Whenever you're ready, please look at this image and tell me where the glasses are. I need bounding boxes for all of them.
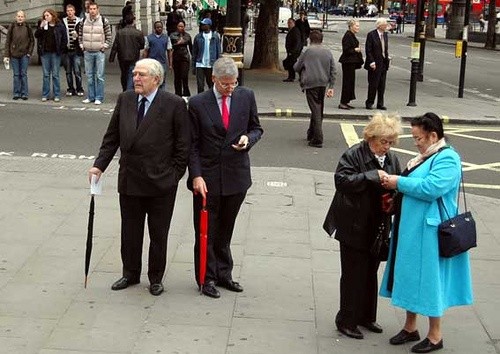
[411,133,431,143]
[217,76,239,89]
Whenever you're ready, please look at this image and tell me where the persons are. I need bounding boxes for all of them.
[79,0,94,21]
[296,12,310,46]
[60,3,85,95]
[108,13,144,91]
[3,9,34,100]
[323,110,402,338]
[190,17,221,93]
[283,17,302,82]
[142,20,173,91]
[338,18,364,108]
[88,58,192,296]
[479,13,484,31]
[167,5,184,35]
[34,8,67,102]
[378,111,474,353]
[116,5,136,28]
[201,4,226,18]
[78,2,113,104]
[169,20,193,103]
[293,29,336,147]
[432,7,450,28]
[166,1,171,10]
[389,11,402,33]
[187,55,264,298]
[122,0,131,14]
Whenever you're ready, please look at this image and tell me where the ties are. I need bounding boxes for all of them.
[380,34,385,58]
[220,94,229,129]
[135,97,146,129]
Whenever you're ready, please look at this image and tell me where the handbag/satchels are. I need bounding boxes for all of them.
[370,214,390,262]
[282,56,297,70]
[437,211,477,258]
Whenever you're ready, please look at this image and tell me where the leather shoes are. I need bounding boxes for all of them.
[197,280,221,299]
[338,103,350,110]
[377,105,387,111]
[346,104,356,109]
[149,279,164,296]
[411,339,444,354]
[360,319,383,335]
[214,277,244,293]
[389,328,421,345]
[110,276,141,290]
[366,104,374,110]
[335,317,364,340]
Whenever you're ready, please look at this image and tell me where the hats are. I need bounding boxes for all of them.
[199,18,212,26]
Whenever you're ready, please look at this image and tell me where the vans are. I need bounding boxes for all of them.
[278,8,292,32]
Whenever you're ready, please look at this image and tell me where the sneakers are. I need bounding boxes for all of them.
[21,97,28,101]
[94,99,102,105]
[308,140,323,148]
[283,77,294,83]
[41,96,49,101]
[77,90,84,97]
[53,96,60,102]
[81,96,94,103]
[13,95,20,100]
[65,90,74,96]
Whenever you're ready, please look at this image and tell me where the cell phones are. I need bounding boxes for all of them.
[236,143,245,147]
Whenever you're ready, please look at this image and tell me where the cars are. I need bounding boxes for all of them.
[307,17,323,32]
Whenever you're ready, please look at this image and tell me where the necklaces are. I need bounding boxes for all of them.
[364,16,389,109]
